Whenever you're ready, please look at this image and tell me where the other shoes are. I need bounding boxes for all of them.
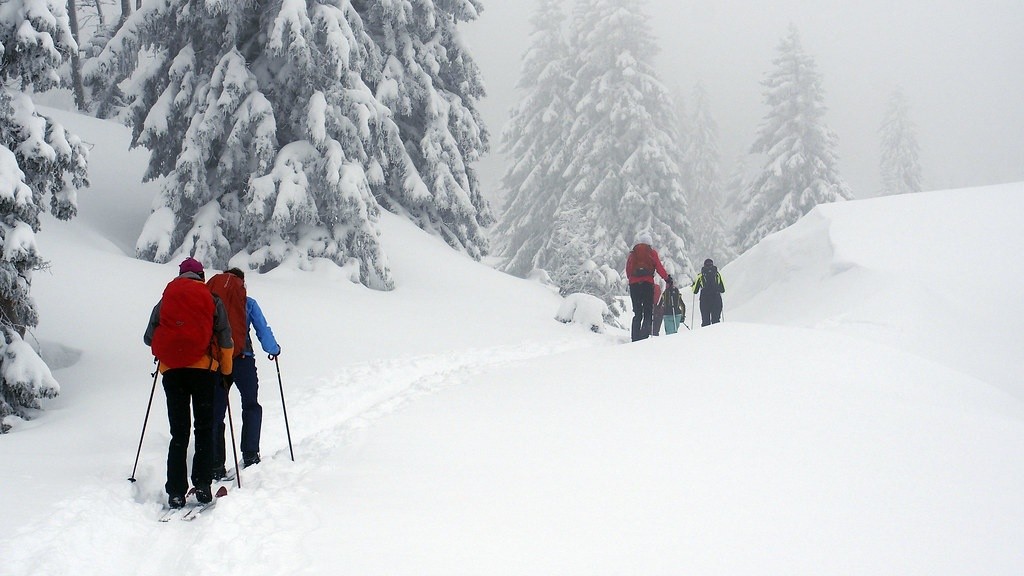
[242,452,259,466]
[213,468,226,480]
[168,493,186,509]
[195,486,212,503]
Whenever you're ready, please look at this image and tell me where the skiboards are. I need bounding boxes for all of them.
[158,485,228,522]
[213,442,261,481]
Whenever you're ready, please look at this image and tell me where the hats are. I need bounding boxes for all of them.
[180,257,204,273]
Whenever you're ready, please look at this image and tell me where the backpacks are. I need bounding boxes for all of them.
[631,242,653,276]
[150,277,215,369]
[701,264,719,294]
[210,273,249,360]
[663,287,680,315]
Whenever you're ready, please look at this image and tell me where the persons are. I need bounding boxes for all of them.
[653,283,683,336]
[144,259,234,508]
[627,236,673,342]
[692,259,725,327]
[212,268,281,480]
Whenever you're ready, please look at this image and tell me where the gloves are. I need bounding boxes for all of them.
[219,342,234,376]
[271,344,281,355]
